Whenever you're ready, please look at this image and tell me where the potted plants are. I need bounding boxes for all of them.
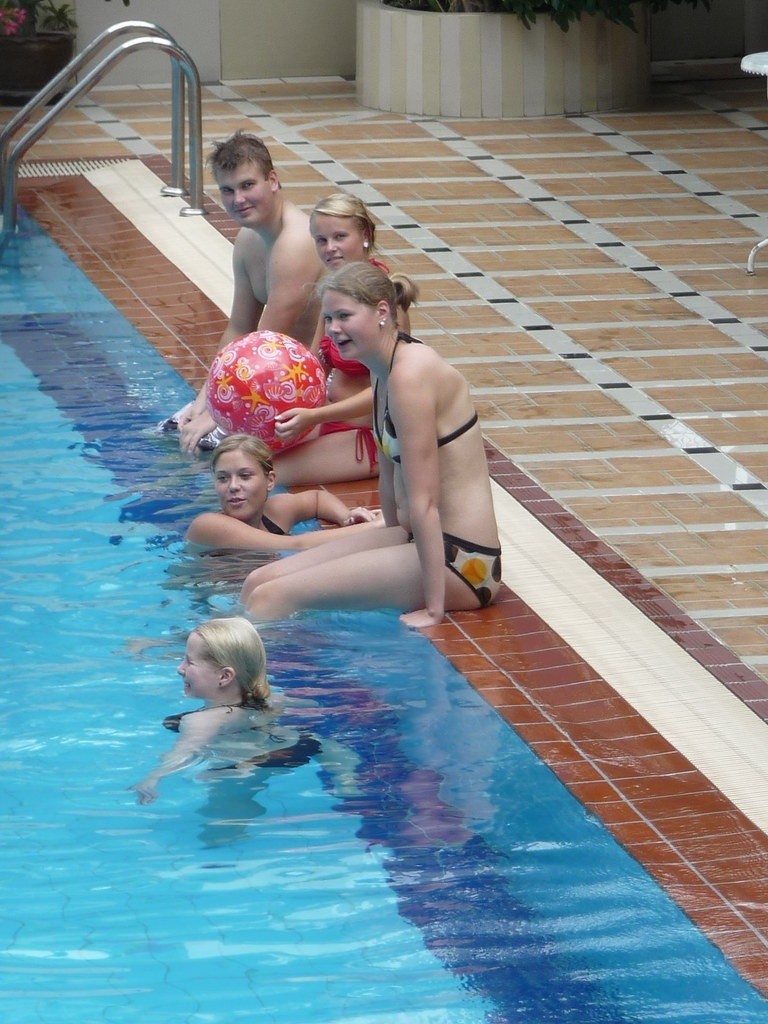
[353,0,669,119]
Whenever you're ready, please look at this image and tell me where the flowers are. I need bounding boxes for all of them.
[0,0,77,37]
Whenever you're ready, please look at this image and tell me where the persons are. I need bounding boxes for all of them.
[184,434,382,554]
[161,129,332,455]
[241,262,503,629]
[206,193,412,486]
[128,617,368,849]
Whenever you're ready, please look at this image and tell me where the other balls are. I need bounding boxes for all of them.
[207,331,331,450]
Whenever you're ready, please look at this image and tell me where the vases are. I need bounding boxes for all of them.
[0,33,75,106]
